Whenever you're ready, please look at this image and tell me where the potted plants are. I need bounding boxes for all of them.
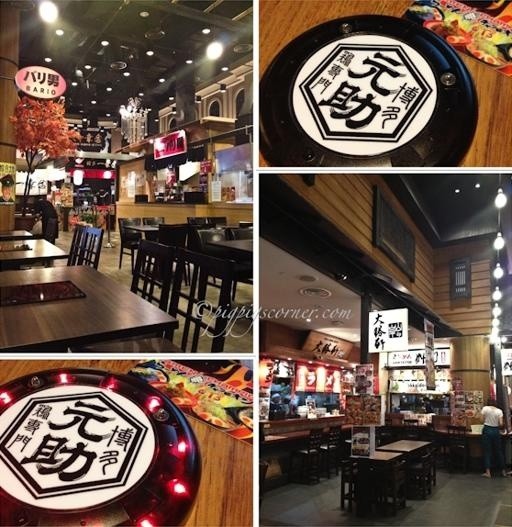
[8,96,83,233]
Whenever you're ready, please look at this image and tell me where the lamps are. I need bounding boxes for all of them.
[206,10,224,60]
[491,260,504,344]
[494,186,508,209]
[119,96,149,147]
[493,230,504,251]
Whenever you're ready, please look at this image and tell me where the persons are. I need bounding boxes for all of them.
[270,393,287,420]
[423,398,434,413]
[0,175,15,203]
[32,196,59,245]
[479,399,507,478]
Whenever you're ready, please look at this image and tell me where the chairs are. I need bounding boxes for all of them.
[0,217,104,272]
[340,420,438,519]
[80,248,235,353]
[288,427,324,485]
[118,217,252,319]
[446,425,469,474]
[309,424,342,480]
[79,237,176,352]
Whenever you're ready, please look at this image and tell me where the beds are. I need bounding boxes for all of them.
[0,266,179,352]
